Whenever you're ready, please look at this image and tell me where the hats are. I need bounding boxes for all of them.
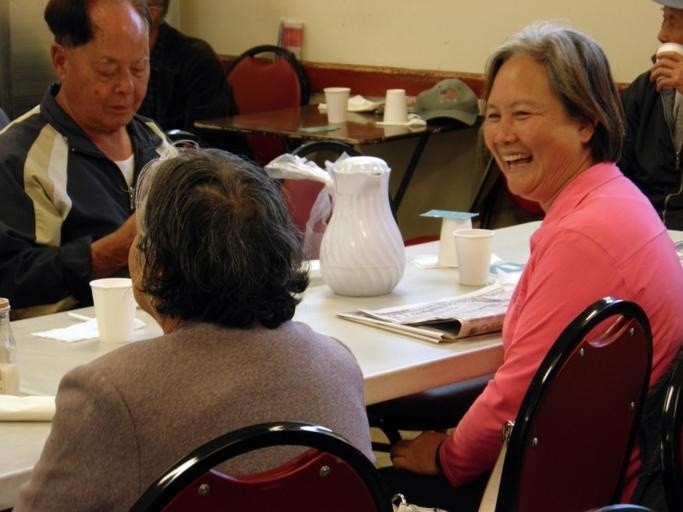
[415,78,480,126]
[652,0,682,10]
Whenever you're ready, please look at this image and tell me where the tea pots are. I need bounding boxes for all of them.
[316,162,404,293]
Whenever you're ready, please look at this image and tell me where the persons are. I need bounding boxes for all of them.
[0,0,181,316]
[377,23,683,512]
[616,0,683,231]
[136,0,260,161]
[11,148,377,512]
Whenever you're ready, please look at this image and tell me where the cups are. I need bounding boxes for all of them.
[325,87,350,125]
[384,89,409,123]
[90,277,136,342]
[438,217,471,269]
[656,42,682,61]
[455,229,496,286]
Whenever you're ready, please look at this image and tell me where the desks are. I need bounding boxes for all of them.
[188,107,483,218]
[1,220,683,512]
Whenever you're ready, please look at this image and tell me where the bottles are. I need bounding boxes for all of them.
[0,297,20,391]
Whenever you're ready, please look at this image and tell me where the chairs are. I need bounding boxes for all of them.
[275,141,360,230]
[631,355,683,512]
[228,46,310,113]
[390,116,504,246]
[393,298,653,512]
[664,181,683,230]
[129,422,394,512]
